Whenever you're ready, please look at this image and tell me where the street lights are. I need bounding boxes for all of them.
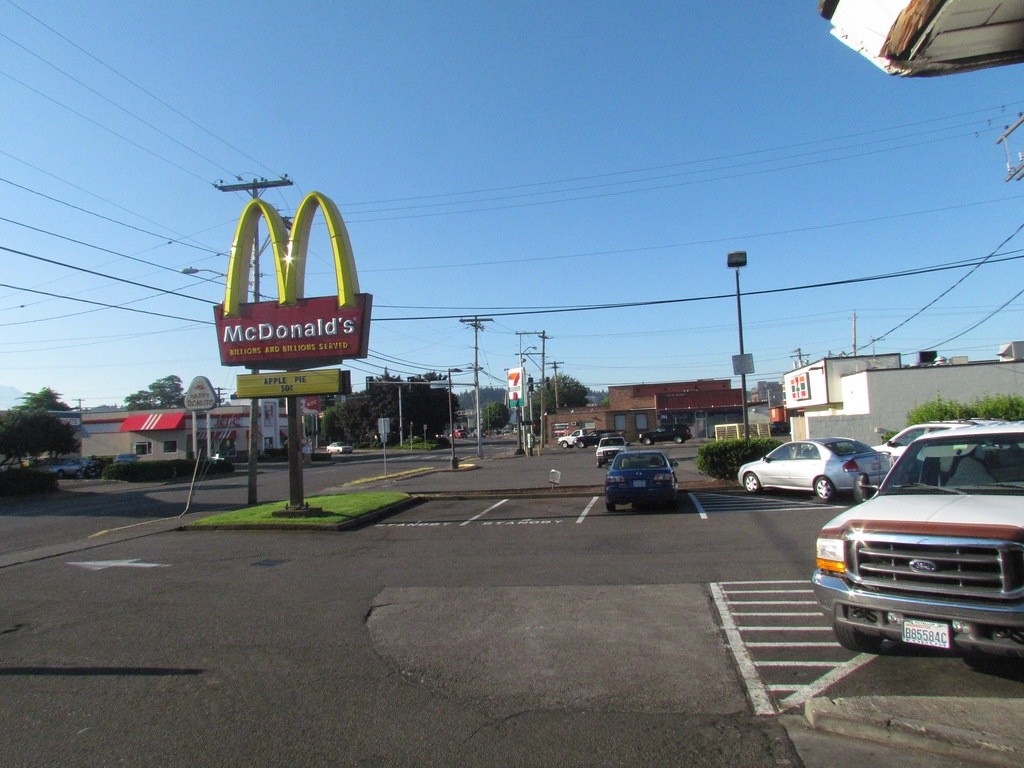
[727,251,749,440]
[448,368,464,469]
[181,266,261,505]
[520,345,538,455]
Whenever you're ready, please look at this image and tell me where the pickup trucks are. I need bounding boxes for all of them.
[557,429,586,449]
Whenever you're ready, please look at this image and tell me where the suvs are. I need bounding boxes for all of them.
[638,423,692,446]
[871,417,1024,464]
[811,425,1024,660]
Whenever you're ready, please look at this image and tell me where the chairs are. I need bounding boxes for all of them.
[945,456,997,486]
[799,448,809,458]
[622,458,629,468]
[650,457,659,468]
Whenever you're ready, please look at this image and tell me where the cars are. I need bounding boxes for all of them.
[593,436,630,469]
[575,429,621,449]
[604,450,679,513]
[24,457,90,480]
[741,437,892,504]
[113,454,141,464]
